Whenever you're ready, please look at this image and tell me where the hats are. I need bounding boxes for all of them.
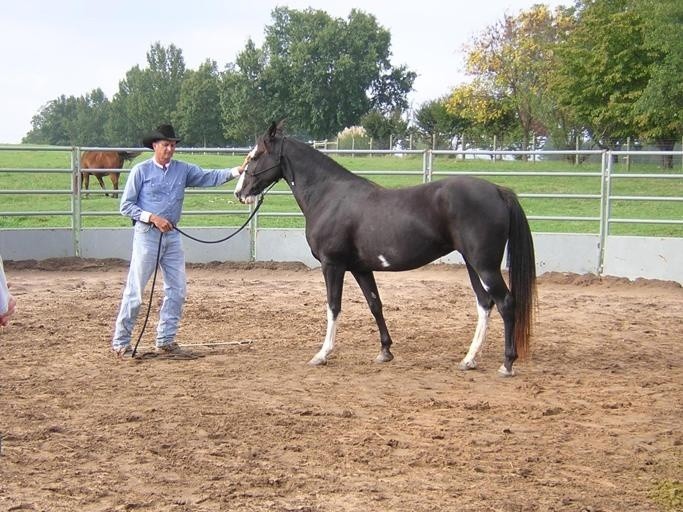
[141,122,182,150]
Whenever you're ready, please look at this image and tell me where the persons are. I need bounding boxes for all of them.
[113,124,251,363]
[0,256,18,328]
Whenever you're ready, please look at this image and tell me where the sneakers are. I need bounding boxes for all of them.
[113,344,142,361]
[153,340,181,354]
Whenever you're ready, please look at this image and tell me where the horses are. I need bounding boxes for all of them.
[233,117,538,378]
[75,150,142,198]
